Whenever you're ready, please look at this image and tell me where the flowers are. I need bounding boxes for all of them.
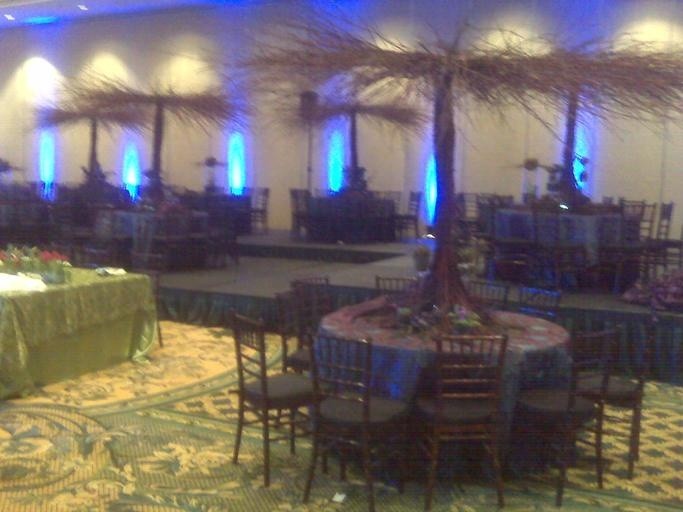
[386,298,481,335]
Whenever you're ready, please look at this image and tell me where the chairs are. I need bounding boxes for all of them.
[515,282,564,323]
[301,330,406,511]
[416,333,510,511]
[0,181,683,306]
[131,265,166,349]
[517,321,624,507]
[271,282,314,428]
[222,306,313,486]
[291,274,332,335]
[574,304,659,482]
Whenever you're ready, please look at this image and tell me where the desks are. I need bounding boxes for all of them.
[0,254,151,399]
